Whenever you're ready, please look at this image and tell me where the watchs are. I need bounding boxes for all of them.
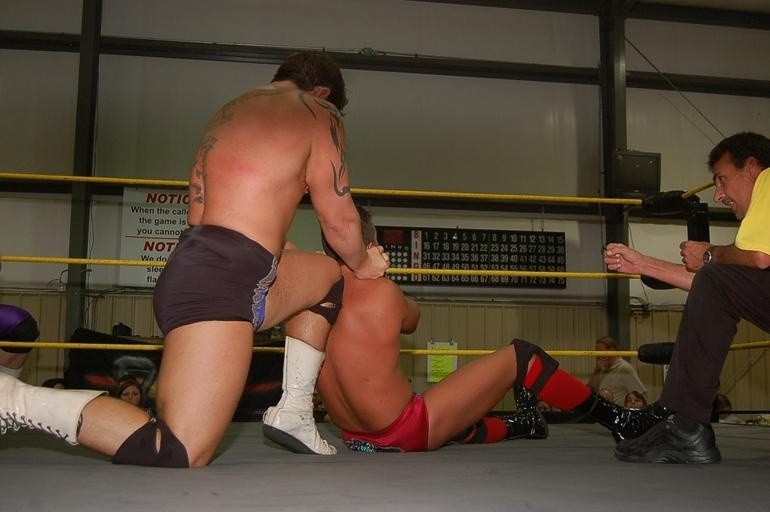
[704,245,718,263]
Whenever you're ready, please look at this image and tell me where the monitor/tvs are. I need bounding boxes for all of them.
[611,149,662,197]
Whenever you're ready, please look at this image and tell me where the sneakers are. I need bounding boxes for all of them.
[612,422,723,466]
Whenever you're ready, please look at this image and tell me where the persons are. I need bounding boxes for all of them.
[590,337,647,409]
[718,394,731,412]
[117,380,145,406]
[604,133,768,464]
[315,206,673,452]
[1,49,390,467]
[43,379,65,389]
[537,400,552,413]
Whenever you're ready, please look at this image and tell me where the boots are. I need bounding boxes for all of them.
[0,372,109,447]
[502,384,550,440]
[576,383,672,445]
[261,336,338,456]
